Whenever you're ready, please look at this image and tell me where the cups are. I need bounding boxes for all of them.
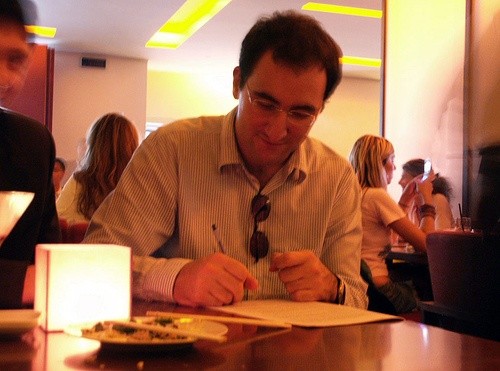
[456,217,472,232]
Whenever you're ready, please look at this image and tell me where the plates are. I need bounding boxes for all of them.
[0,309,40,337]
[82,314,228,348]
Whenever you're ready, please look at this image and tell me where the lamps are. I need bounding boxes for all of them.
[33,244,131,332]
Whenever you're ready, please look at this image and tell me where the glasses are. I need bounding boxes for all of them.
[244,82,322,130]
[250,192,272,263]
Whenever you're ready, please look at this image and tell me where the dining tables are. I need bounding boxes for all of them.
[0,296,500,371]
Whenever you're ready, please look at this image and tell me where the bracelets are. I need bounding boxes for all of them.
[419,204,436,221]
[399,202,410,208]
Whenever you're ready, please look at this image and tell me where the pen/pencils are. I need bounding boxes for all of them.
[211,223,244,304]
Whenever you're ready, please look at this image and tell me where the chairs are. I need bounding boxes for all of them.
[420,233,500,342]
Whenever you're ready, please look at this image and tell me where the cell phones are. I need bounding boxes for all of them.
[421,158,432,182]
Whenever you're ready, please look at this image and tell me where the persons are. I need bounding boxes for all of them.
[56,112,138,225]
[0,0,61,309]
[80,10,369,310]
[349,134,436,314]
[396,159,456,242]
[52,158,65,200]
